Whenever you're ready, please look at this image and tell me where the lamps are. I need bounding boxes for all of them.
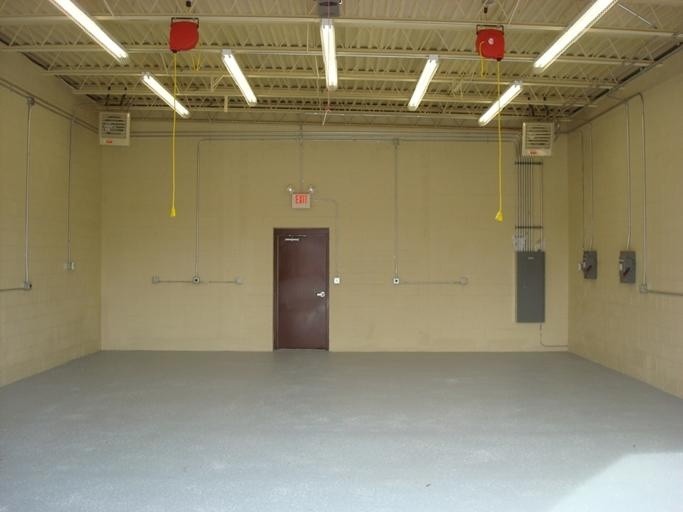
[219,50,257,107]
[51,1,129,67]
[531,0,616,76]
[139,73,191,119]
[319,17,338,93]
[406,56,440,112]
[477,81,522,125]
